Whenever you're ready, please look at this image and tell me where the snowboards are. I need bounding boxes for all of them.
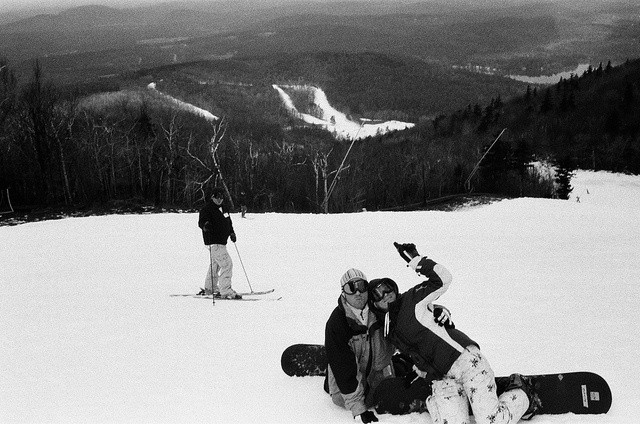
[374,372,612,414]
[281,344,328,377]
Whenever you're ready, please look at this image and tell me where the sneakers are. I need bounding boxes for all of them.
[219,291,237,300]
[505,374,544,421]
[205,289,220,295]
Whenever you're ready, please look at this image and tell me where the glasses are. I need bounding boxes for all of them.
[341,279,372,295]
[369,281,393,303]
[212,193,224,200]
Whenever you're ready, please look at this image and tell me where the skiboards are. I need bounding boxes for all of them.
[169,289,282,301]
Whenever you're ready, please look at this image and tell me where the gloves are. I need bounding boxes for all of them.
[354,409,379,424]
[205,223,212,231]
[428,303,454,327]
[230,233,236,242]
[393,241,421,264]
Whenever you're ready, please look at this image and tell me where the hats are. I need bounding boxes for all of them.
[340,268,369,287]
[370,277,399,303]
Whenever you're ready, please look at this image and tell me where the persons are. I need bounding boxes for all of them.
[575,195,582,203]
[323,268,398,424]
[368,241,543,424]
[240,192,249,220]
[198,189,243,300]
